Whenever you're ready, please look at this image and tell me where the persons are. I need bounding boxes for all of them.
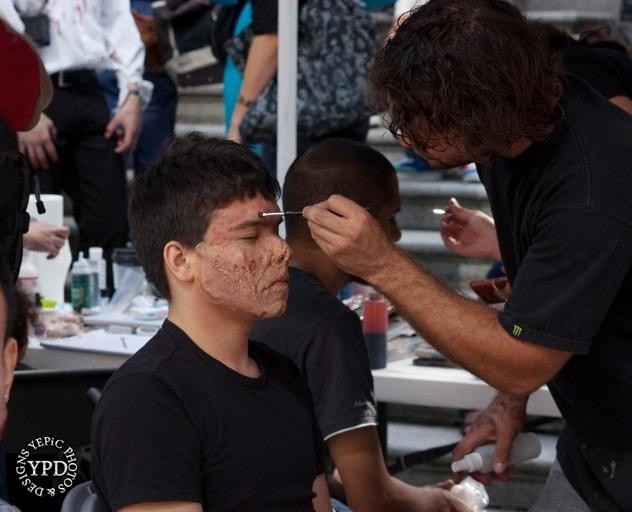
[1,1,632,511]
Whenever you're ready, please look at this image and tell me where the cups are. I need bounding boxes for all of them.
[18,264,42,337]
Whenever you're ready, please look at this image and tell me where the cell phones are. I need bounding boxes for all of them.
[471,277,509,304]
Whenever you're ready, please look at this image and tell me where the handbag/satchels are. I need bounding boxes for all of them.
[225,0,387,144]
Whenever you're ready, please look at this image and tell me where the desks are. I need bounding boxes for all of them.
[371,355,565,478]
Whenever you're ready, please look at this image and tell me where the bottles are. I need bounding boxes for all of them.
[71,246,107,316]
[362,292,388,370]
[451,432,541,475]
